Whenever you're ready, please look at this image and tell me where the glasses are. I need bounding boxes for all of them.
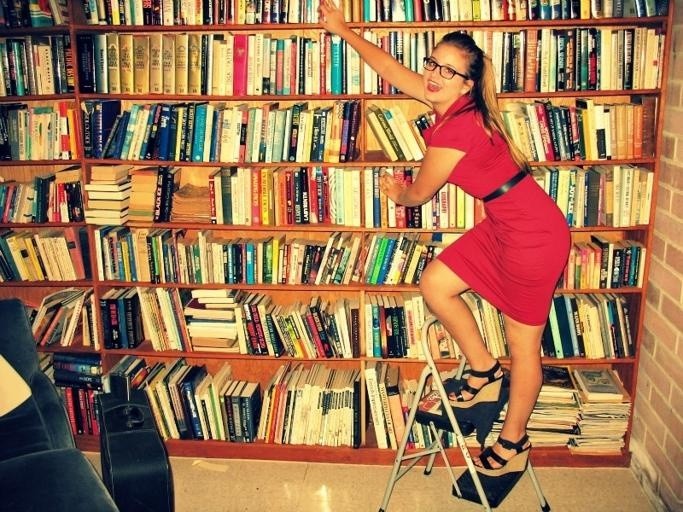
[423,57,470,81]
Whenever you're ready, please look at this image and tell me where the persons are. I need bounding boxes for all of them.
[317,0,571,477]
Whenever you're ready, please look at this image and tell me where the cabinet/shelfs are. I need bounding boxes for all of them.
[0,0,675,469]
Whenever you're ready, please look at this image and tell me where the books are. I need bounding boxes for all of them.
[92,226,467,285]
[365,104,438,162]
[362,26,666,97]
[556,234,646,289]
[23,286,100,351]
[525,163,654,229]
[0,0,70,29]
[0,225,94,281]
[0,103,79,161]
[364,293,510,361]
[0,162,85,225]
[540,293,634,360]
[98,286,359,360]
[364,0,674,22]
[366,361,633,457]
[362,164,486,232]
[37,351,103,435]
[84,164,362,226]
[0,31,76,98]
[76,28,364,97]
[73,0,361,26]
[80,99,362,165]
[501,94,655,162]
[107,355,360,449]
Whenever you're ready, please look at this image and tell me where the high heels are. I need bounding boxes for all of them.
[447,360,503,408]
[472,435,532,476]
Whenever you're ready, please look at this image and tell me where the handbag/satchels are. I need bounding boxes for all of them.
[97,387,176,512]
